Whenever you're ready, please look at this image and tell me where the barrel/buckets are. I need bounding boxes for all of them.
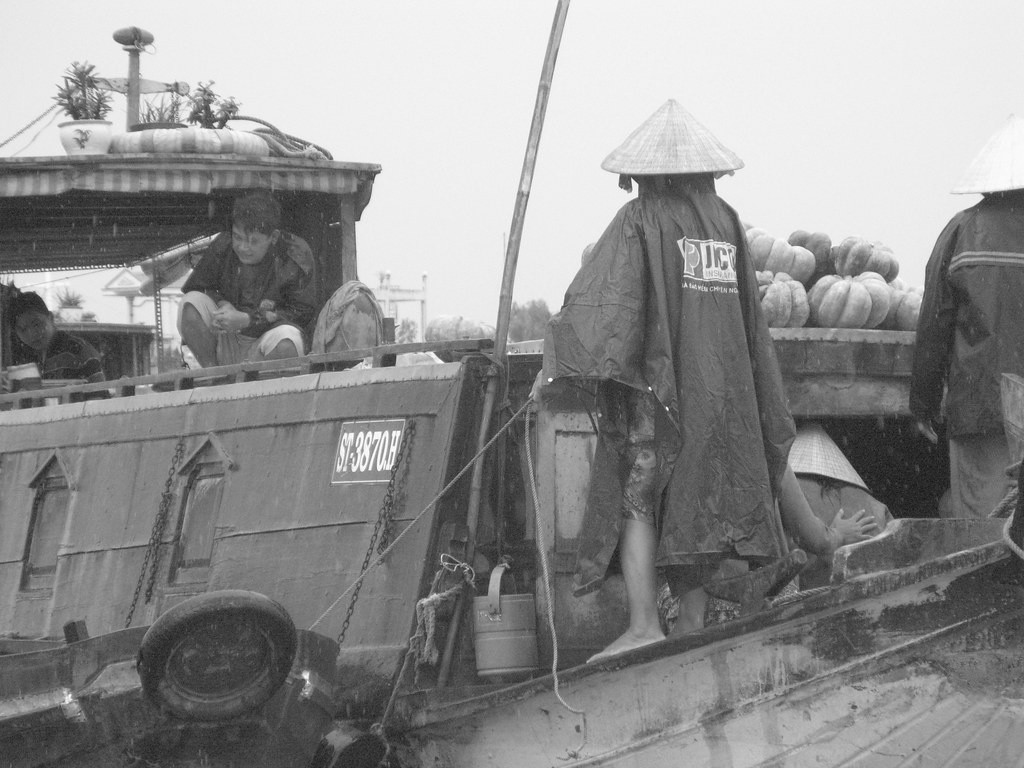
[470,562,540,677]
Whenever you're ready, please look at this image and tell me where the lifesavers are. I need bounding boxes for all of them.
[110,125,271,157]
[136,588,298,722]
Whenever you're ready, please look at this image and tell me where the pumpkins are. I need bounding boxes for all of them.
[506,294,556,343]
[742,226,923,330]
[423,316,497,362]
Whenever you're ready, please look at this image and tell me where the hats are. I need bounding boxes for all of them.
[950,109,1024,195]
[600,99,745,175]
[788,422,874,494]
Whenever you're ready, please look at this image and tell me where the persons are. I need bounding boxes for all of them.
[909,155,1024,519]
[657,421,896,637]
[0,291,111,407]
[176,192,320,388]
[527,99,797,665]
